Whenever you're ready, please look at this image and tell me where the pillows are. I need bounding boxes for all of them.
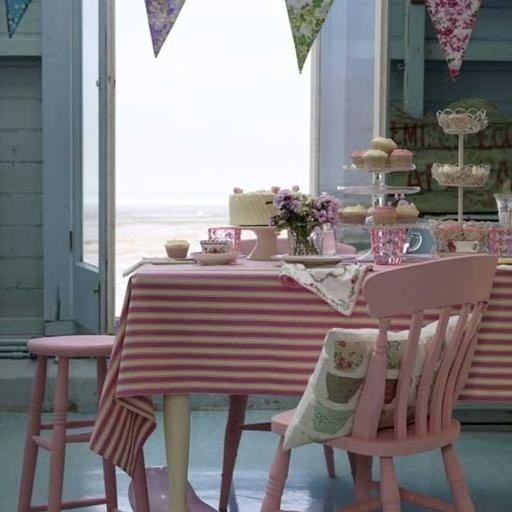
[282,312,477,451]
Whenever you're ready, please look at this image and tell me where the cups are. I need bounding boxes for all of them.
[448,240,479,253]
[201,240,229,253]
[370,227,422,265]
[320,229,336,255]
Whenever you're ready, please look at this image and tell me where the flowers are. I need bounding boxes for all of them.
[266,186,341,255]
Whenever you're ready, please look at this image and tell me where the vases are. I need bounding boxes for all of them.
[494,190,512,236]
[286,222,326,257]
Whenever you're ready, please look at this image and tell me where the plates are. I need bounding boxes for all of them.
[437,108,486,135]
[232,225,287,261]
[433,162,490,187]
[190,251,238,265]
[429,219,493,252]
[343,164,416,172]
[272,253,355,267]
[337,220,432,229]
[337,186,420,195]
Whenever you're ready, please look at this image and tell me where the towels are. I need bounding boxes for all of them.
[278,263,373,316]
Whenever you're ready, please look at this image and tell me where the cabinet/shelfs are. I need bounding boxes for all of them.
[374,3,512,219]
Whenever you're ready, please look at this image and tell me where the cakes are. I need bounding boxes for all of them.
[371,137,399,159]
[229,186,299,225]
[389,149,413,169]
[344,204,367,224]
[362,149,387,169]
[366,206,373,216]
[350,149,365,167]
[337,208,349,224]
[396,199,420,224]
[374,207,397,227]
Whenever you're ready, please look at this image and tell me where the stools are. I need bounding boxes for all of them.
[17,334,122,508]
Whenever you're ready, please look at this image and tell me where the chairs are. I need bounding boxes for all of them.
[256,253,500,507]
